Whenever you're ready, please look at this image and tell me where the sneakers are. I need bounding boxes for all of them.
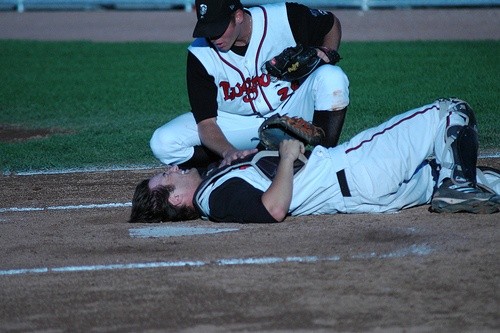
[431,176,500,214]
[476,166,500,196]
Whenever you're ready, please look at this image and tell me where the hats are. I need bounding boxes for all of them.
[193,0,240,39]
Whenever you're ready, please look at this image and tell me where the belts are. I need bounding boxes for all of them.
[336,169,352,197]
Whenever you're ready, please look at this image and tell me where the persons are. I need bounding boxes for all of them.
[150,0,350,178]
[128,95,500,223]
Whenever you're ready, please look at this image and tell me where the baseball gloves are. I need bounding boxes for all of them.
[258,113,325,150]
[265,44,344,82]
